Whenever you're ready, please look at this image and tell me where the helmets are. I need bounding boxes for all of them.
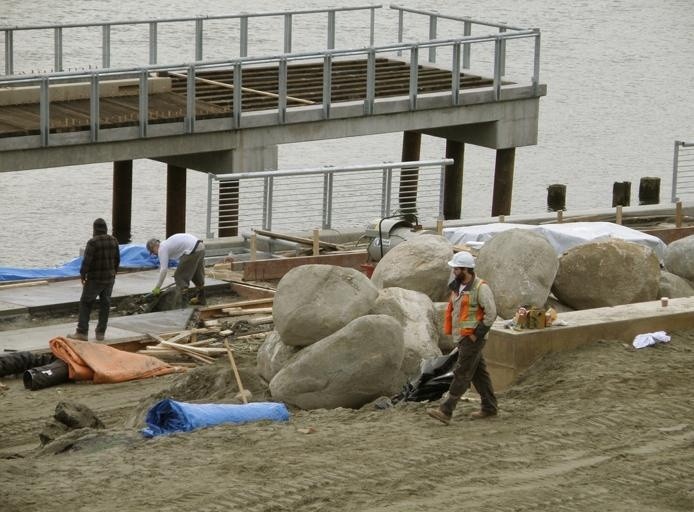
[448,251,475,268]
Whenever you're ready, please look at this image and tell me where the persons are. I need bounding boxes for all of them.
[66,217,120,342]
[146,233,207,306]
[424,251,499,426]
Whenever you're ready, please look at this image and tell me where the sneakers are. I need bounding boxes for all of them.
[428,409,450,425]
[66,333,88,341]
[471,411,497,419]
[96,334,104,340]
[189,298,206,305]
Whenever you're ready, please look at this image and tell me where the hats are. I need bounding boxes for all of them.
[147,239,158,255]
